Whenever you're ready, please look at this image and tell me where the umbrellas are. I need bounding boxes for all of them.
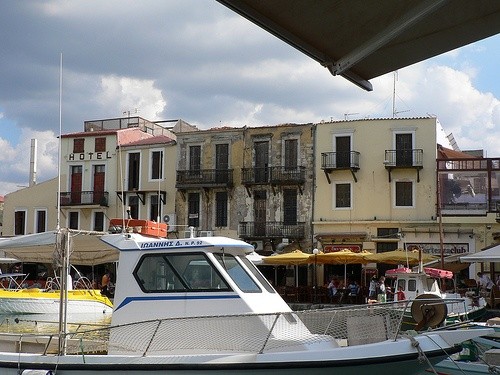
[308,248,373,306]
[188,251,270,290]
[364,247,440,272]
[261,250,315,302]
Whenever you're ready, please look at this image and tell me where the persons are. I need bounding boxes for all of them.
[477,272,497,292]
[101,270,114,294]
[378,274,393,303]
[327,279,362,304]
[369,272,378,302]
[391,285,406,300]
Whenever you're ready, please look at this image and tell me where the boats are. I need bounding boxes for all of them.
[0,53,499,375]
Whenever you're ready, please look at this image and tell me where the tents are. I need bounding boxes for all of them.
[460,244,499,263]
